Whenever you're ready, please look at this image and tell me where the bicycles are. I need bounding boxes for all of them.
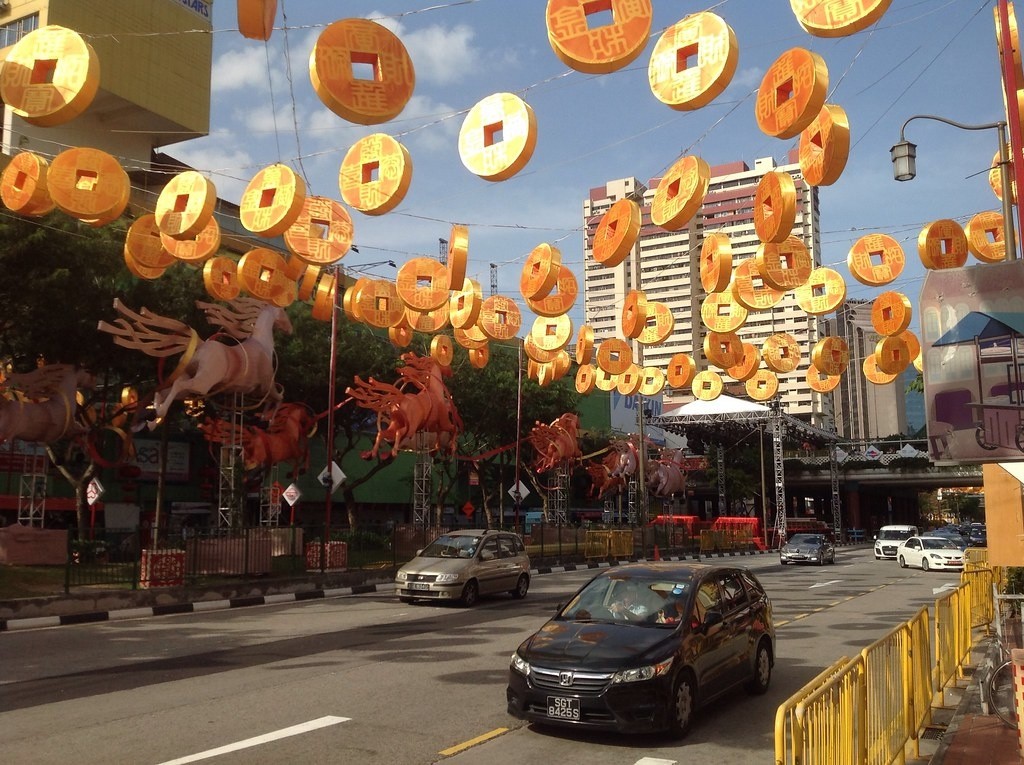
[986,657,1019,731]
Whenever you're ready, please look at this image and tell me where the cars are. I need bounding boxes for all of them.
[926,522,987,547]
[778,533,835,566]
[896,535,964,572]
[505,560,777,741]
[393,527,533,603]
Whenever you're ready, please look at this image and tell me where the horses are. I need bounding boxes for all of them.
[1,296,687,503]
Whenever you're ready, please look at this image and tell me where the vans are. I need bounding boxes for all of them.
[873,524,919,562]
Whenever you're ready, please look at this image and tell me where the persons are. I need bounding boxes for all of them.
[656,593,700,628]
[607,583,648,622]
